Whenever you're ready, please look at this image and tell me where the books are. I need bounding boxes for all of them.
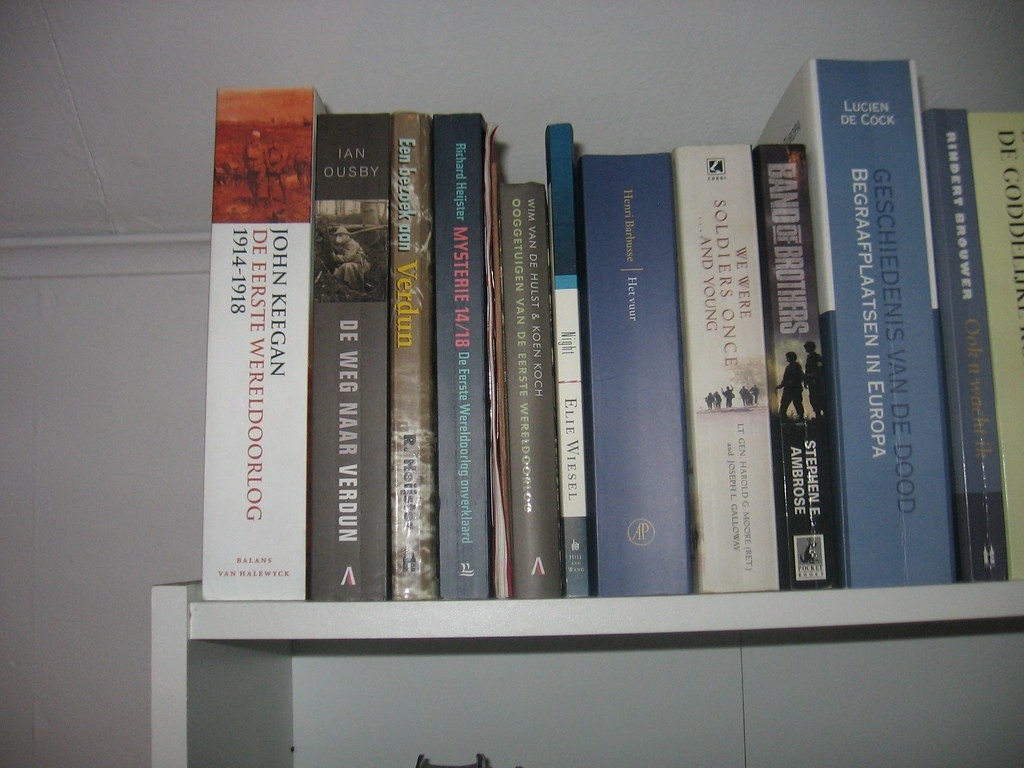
[198,53,1024,599]
